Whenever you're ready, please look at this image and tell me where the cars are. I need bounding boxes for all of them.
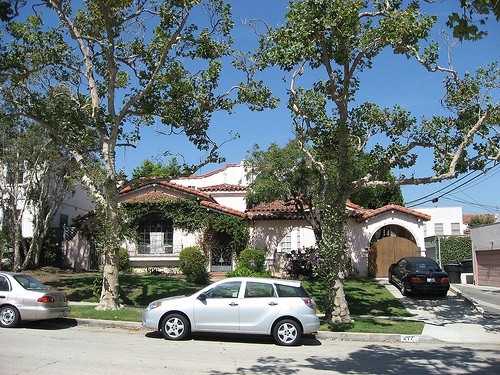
[388,256,450,296]
[142,276,321,345]
[0,272,71,328]
